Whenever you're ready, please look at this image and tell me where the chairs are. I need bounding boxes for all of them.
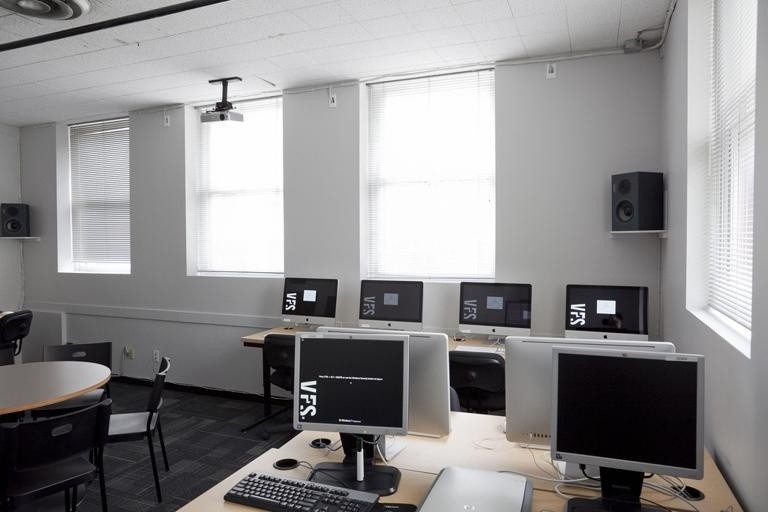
[0,306,178,511]
[254,332,304,443]
[443,346,509,418]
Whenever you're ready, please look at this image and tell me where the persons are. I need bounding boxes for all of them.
[602,313,625,330]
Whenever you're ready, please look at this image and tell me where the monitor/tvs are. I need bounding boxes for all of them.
[293,332,410,494]
[550,343,705,512]
[318,327,450,463]
[565,283,649,341]
[505,336,676,483]
[458,281,532,339]
[281,276,340,328]
[358,279,424,332]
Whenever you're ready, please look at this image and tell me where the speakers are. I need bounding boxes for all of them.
[0,203,29,237]
[611,171,663,231]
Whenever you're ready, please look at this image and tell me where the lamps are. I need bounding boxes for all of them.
[0,1,93,21]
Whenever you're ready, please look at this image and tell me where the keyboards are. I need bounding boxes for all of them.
[222,472,379,512]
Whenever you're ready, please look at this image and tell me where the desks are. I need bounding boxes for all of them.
[273,407,747,512]
[234,323,507,435]
[173,446,751,511]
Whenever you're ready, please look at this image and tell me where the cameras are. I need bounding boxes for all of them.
[601,317,615,326]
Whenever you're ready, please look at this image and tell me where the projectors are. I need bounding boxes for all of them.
[200,110,244,123]
